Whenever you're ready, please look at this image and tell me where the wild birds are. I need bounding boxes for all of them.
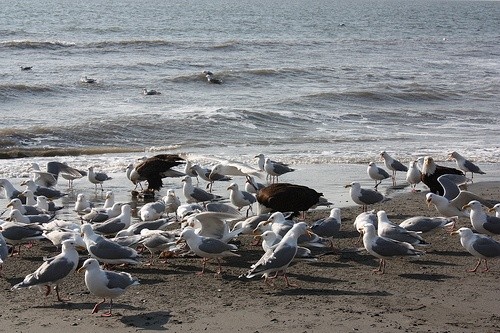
[0,66,500,317]
